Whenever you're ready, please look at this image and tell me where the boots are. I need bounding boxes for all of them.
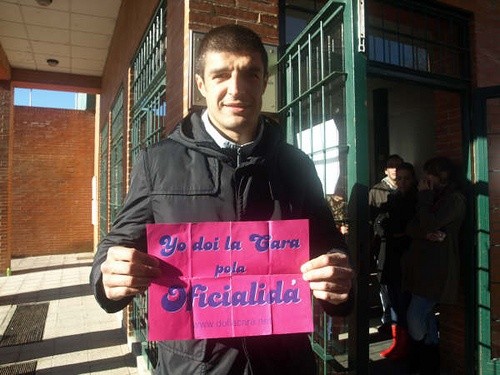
[379,324,440,375]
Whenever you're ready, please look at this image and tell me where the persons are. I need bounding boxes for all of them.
[368,155,474,362]
[90,24,356,375]
